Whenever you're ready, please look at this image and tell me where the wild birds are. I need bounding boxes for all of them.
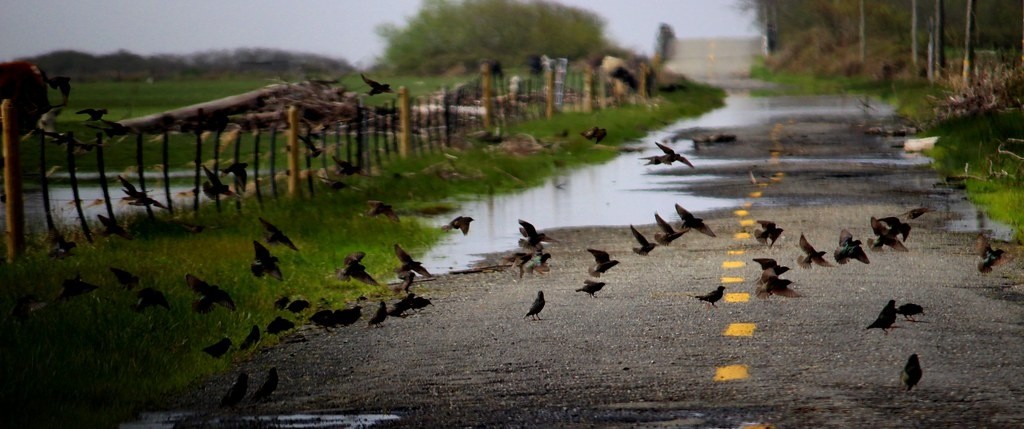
[5,52,1009,414]
